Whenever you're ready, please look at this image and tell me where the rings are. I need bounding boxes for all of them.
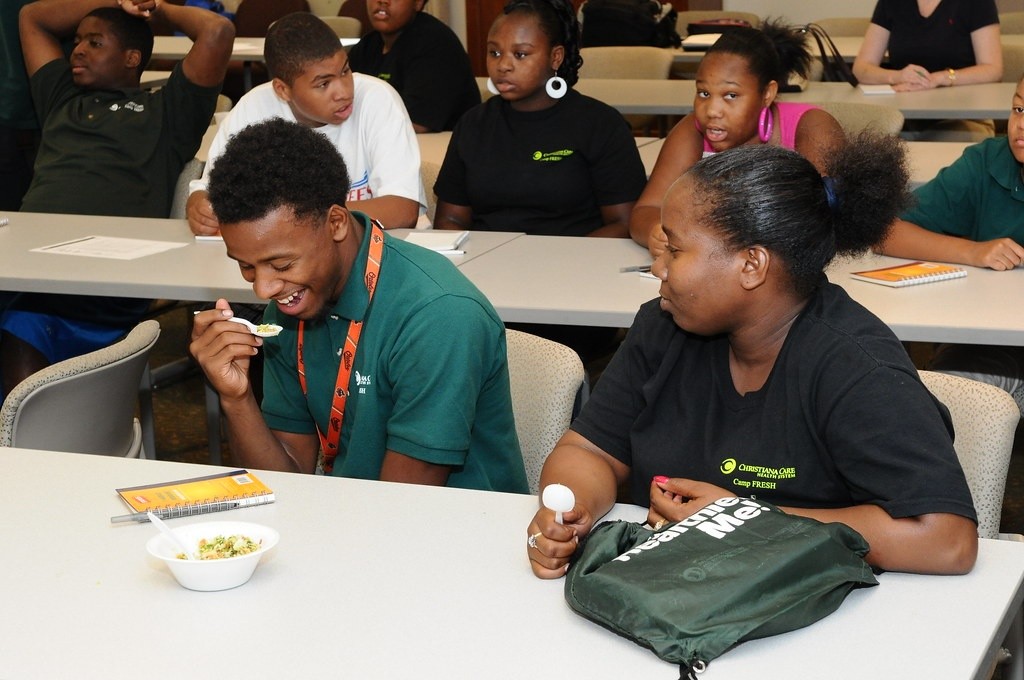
[528,533,542,550]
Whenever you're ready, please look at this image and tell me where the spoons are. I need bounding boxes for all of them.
[194,310,283,336]
[543,483,574,524]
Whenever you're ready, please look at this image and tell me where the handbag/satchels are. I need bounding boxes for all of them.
[806,19,856,87]
[565,495,880,673]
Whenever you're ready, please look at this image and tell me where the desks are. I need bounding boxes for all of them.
[0,35,1024,680]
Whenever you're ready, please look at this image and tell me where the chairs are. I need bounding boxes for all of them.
[169,93,233,221]
[503,326,589,495]
[676,9,761,40]
[804,17,870,83]
[805,96,909,144]
[916,369,1020,539]
[0,320,161,458]
[575,46,674,137]
[321,18,362,53]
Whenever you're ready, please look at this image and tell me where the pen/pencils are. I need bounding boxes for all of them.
[437,250,466,254]
[110,502,241,522]
[621,264,652,273]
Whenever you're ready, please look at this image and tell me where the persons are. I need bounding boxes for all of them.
[872,73,1024,272]
[851,0,1004,145]
[627,17,850,260]
[432,1,647,239]
[344,0,484,136]
[0,1,236,407]
[526,121,977,580]
[188,117,531,495]
[185,12,429,235]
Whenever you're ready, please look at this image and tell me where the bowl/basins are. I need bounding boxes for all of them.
[146,522,280,591]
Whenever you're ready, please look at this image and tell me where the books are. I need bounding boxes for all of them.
[117,468,276,525]
[849,262,968,288]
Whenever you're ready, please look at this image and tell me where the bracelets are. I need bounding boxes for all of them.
[945,68,955,87]
